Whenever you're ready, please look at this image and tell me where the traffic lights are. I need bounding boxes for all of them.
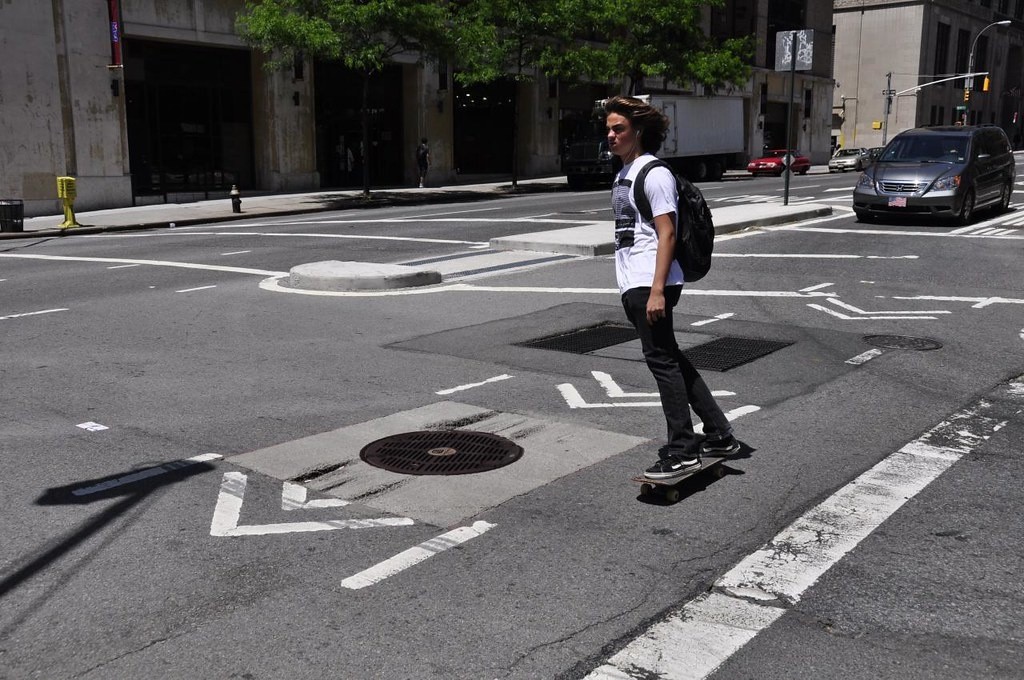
[964,89,969,104]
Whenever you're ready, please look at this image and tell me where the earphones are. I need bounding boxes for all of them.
[636,130,640,135]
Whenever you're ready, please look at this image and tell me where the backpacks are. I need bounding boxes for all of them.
[633,158,715,281]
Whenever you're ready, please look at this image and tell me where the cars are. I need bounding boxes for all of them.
[746,150,811,177]
[828,146,892,172]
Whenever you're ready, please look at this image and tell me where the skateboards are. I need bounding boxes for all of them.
[630,455,728,502]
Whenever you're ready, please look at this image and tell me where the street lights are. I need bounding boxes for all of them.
[964,21,1011,127]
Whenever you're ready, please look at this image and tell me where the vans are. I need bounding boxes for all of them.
[853,126,1016,226]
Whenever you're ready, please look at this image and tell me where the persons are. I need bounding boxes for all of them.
[415,138,432,188]
[604,96,740,481]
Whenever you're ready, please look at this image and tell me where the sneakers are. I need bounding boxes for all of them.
[644,454,702,481]
[697,436,743,457]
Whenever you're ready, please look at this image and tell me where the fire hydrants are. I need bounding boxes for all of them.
[229,185,242,213]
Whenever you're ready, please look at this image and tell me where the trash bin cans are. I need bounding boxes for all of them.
[0,199,24,233]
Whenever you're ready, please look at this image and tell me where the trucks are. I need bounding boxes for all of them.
[548,94,743,191]
